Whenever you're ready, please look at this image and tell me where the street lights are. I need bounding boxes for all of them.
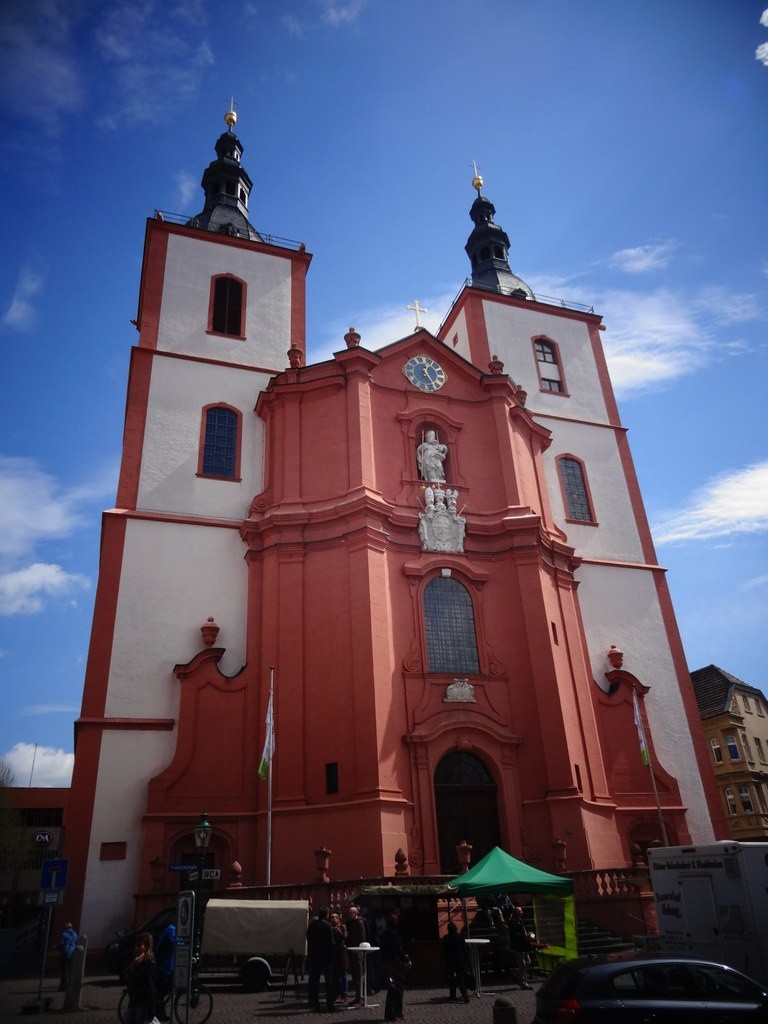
[194,811,214,922]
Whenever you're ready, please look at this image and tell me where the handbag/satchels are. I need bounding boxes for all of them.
[62,955,70,964]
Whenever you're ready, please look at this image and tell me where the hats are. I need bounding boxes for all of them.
[66,923,72,927]
[514,907,523,914]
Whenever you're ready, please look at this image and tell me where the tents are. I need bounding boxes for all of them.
[435,847,581,998]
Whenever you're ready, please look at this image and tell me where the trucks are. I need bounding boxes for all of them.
[106,899,310,984]
[647,839,768,988]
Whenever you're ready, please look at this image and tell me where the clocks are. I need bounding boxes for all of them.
[402,353,449,393]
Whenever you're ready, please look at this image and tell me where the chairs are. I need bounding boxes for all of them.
[641,968,703,998]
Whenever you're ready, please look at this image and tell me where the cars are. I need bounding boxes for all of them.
[530,951,768,1024]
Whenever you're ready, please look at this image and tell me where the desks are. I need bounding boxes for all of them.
[463,939,497,999]
[347,947,379,1010]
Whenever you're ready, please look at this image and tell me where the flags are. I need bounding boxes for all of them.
[257,691,276,783]
[633,691,649,768]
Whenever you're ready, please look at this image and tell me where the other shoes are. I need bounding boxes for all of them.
[326,1006,339,1013]
[515,977,522,986]
[385,1017,406,1024]
[396,1015,406,1019]
[521,983,534,990]
[310,1005,322,1011]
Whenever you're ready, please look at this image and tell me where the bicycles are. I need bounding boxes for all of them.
[118,945,214,1024]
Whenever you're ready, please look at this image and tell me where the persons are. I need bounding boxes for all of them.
[306,906,344,1011]
[442,921,472,1003]
[56,920,78,990]
[509,906,535,991]
[373,904,412,1022]
[346,906,366,1007]
[125,931,163,1024]
[325,909,343,1007]
[689,971,712,1000]
[352,904,381,995]
[417,428,448,482]
[327,913,349,1003]
